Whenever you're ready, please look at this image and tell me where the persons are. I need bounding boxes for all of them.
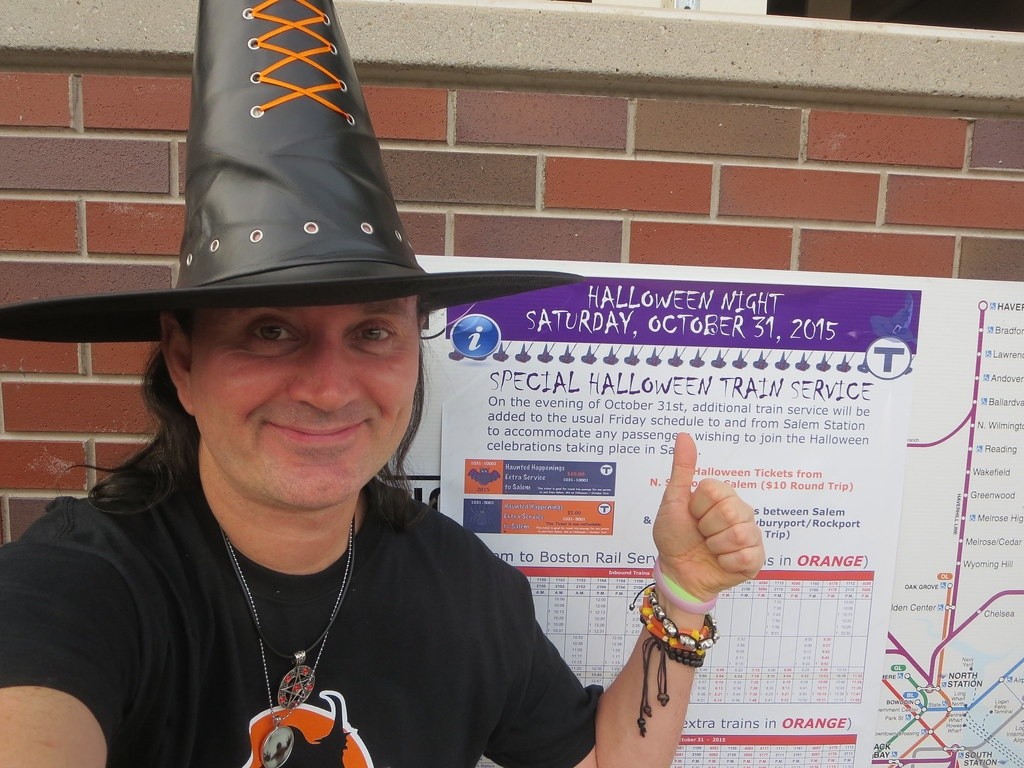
[0,193,764,768]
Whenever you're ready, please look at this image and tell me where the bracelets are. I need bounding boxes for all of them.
[630,556,720,736]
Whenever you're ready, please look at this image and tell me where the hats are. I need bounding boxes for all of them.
[0,0,586,343]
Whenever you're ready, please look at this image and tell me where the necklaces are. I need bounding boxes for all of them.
[225,523,353,768]
[219,516,354,709]
